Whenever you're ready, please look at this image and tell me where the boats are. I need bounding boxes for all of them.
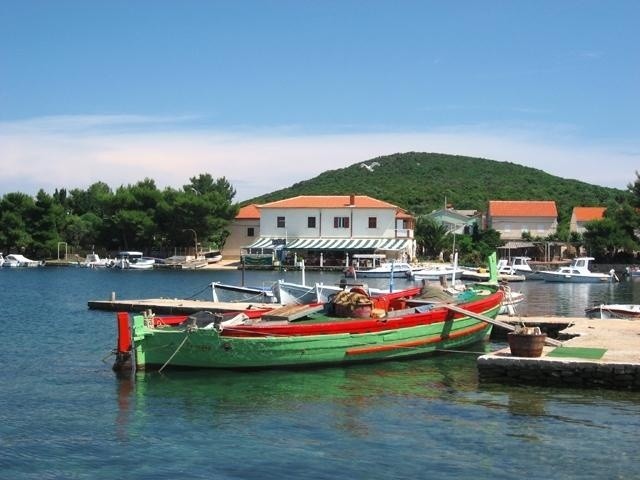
[111,252,155,268]
[540,256,609,284]
[585,304,640,319]
[0,253,42,267]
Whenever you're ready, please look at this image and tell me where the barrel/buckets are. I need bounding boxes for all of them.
[507,327,547,357]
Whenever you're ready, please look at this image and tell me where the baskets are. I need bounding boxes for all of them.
[508,333,547,357]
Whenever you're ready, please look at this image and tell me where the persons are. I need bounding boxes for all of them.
[609,263,640,282]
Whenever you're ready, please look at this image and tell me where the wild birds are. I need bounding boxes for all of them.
[359,161,380,173]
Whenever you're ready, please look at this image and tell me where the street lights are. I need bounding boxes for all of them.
[182,229,197,259]
